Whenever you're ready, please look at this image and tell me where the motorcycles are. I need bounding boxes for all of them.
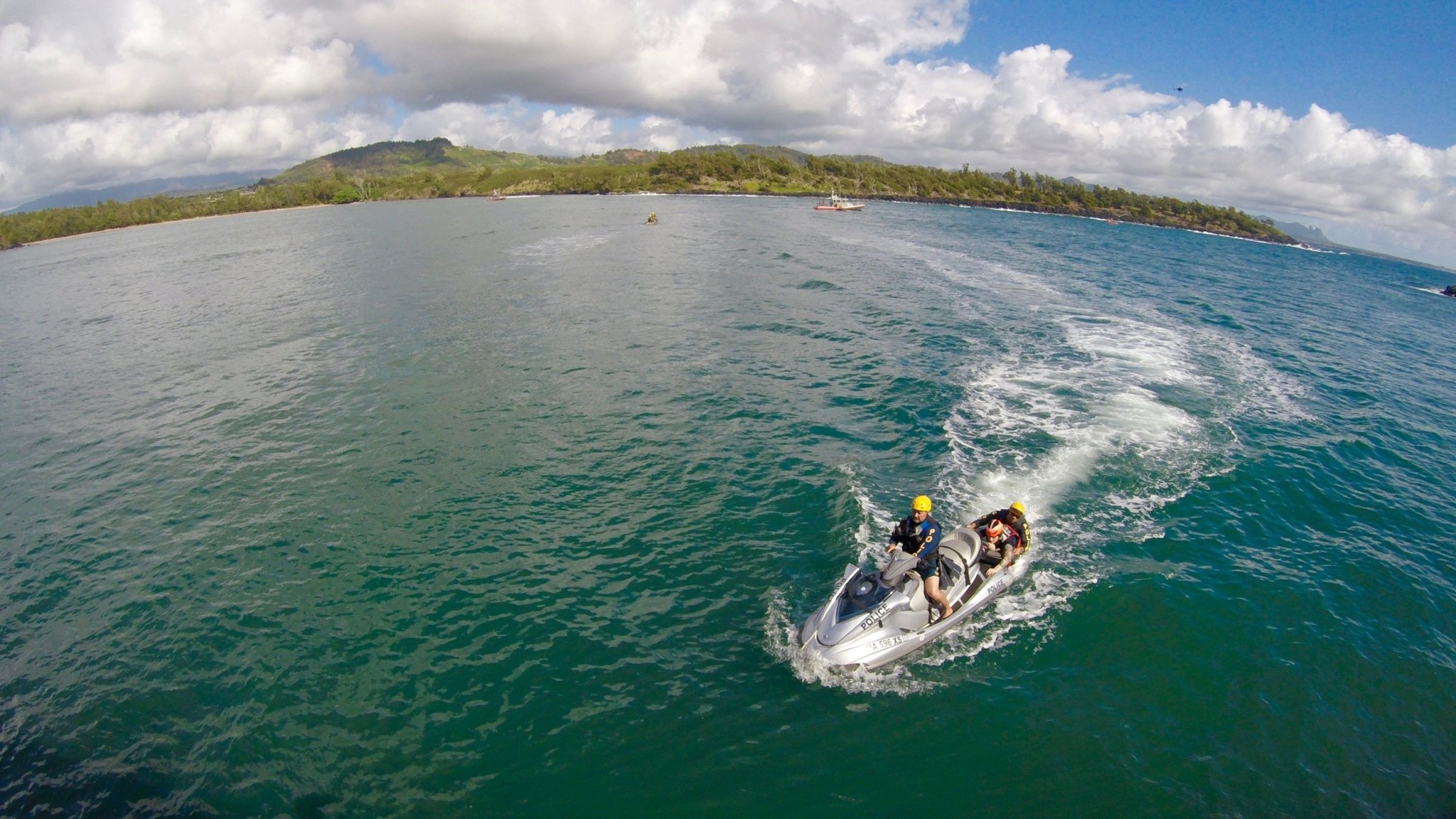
[793,503,1036,676]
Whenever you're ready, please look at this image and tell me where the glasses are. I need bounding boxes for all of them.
[1011,512,1020,519]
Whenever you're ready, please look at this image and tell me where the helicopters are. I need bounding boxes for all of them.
[1173,87,1186,92]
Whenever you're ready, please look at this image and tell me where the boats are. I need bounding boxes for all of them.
[812,190,870,214]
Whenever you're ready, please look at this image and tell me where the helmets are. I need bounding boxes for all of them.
[911,495,932,512]
[1010,502,1024,517]
[986,519,1002,536]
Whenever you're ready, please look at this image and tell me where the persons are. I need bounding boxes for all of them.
[887,495,953,619]
[966,502,1031,576]
